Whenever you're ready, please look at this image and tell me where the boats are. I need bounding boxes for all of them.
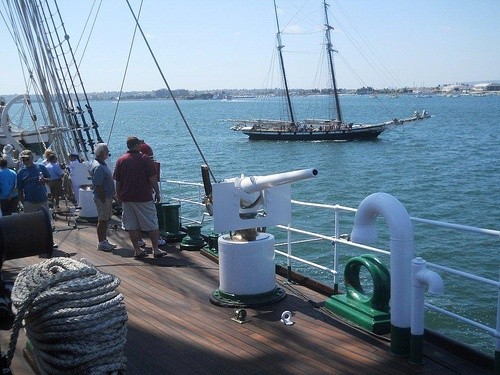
[0,95,57,154]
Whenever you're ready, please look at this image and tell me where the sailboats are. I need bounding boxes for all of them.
[228,0,434,141]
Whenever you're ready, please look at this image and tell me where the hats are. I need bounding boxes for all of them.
[3,144,13,153]
[20,150,32,157]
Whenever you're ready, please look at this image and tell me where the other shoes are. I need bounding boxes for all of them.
[153,249,168,257]
[53,243,58,248]
[134,250,145,260]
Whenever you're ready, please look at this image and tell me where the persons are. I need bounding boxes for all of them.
[90,143,117,251]
[138,143,166,247]
[113,136,168,259]
[0,144,88,247]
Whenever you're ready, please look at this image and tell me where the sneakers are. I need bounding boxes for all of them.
[97,241,117,250]
[138,240,146,247]
[157,240,165,246]
